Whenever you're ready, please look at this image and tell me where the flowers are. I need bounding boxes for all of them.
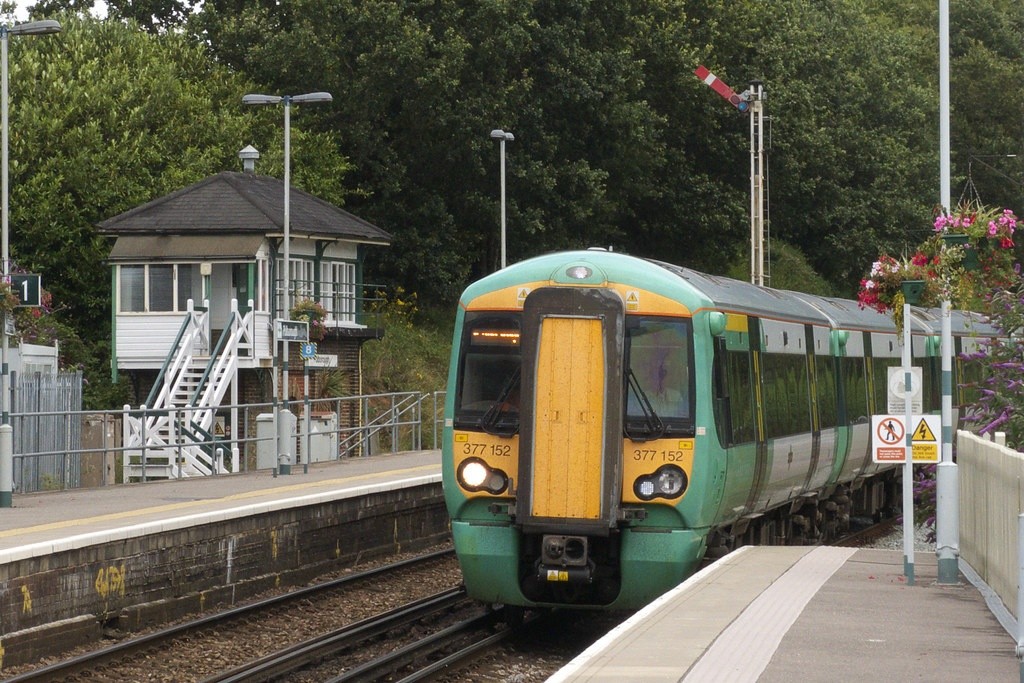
[0,257,52,319]
[857,200,1024,544]
[287,286,419,341]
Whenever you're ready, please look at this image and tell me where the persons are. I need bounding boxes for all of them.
[622,355,687,419]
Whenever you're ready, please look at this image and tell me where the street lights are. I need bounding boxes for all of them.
[240,91,334,475]
[0,20,62,509]
[489,128,515,270]
[898,278,928,588]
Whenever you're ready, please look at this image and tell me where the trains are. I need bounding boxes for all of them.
[442,245,1022,612]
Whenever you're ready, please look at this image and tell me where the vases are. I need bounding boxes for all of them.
[976,236,989,251]
[900,280,926,304]
[940,233,969,249]
[991,238,1001,249]
